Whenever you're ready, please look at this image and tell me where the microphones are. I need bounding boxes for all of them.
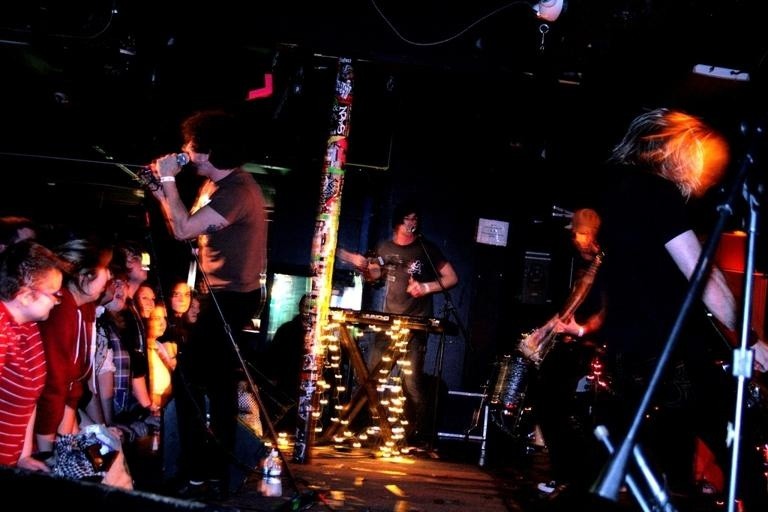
[140,152,189,176]
[409,227,423,239]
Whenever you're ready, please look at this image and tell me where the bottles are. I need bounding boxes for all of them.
[259,474,284,498]
[261,449,282,476]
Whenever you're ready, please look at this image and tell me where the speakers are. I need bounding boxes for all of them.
[520,252,553,305]
[0,466,205,512]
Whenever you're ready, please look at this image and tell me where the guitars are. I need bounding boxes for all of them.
[536,257,600,364]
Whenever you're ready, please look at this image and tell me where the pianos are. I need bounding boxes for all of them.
[328,306,457,336]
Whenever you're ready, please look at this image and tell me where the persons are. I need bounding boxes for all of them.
[1,109,768,512]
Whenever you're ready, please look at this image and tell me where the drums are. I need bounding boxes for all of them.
[487,354,532,407]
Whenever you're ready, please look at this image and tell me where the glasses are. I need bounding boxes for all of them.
[19,282,65,305]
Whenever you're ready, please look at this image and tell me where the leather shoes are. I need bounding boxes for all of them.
[162,481,210,504]
[207,478,226,503]
